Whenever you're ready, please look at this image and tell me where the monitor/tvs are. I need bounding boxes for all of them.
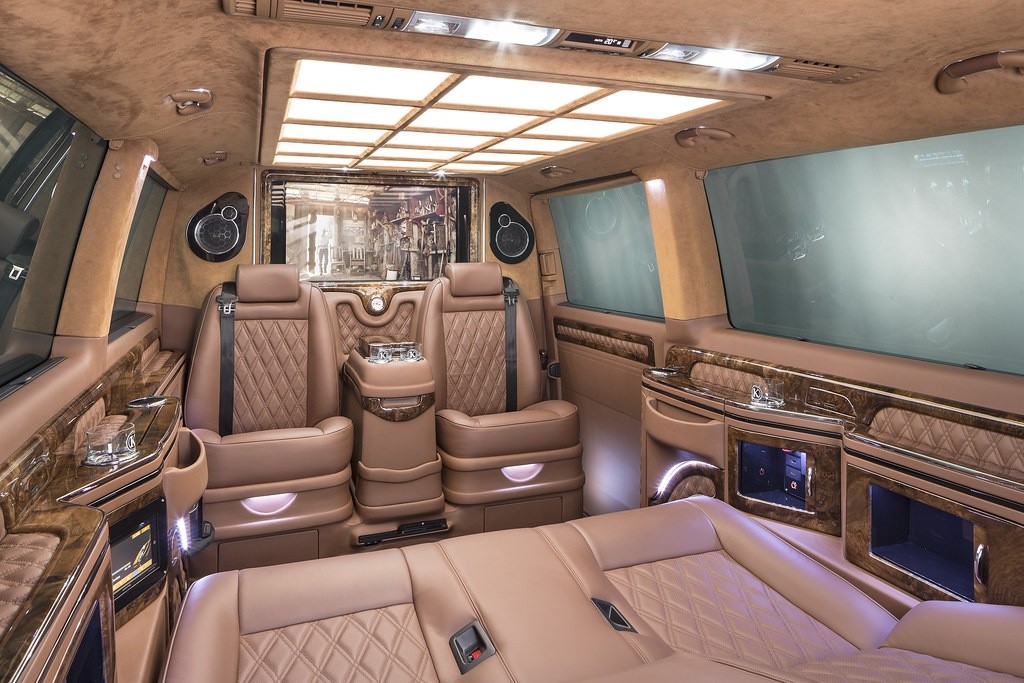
[110,515,159,599]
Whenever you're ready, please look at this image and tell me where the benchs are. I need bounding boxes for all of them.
[156,492,1024,683]
[323,287,424,370]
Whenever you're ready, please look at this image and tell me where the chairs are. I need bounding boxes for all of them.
[419,261,586,506]
[179,262,354,541]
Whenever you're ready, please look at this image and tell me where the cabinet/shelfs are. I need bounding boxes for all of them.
[837,421,1023,606]
[721,390,851,536]
[341,344,446,523]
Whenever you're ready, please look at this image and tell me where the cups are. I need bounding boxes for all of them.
[751,378,785,405]
[400,342,422,362]
[86,423,137,467]
[369,343,393,364]
